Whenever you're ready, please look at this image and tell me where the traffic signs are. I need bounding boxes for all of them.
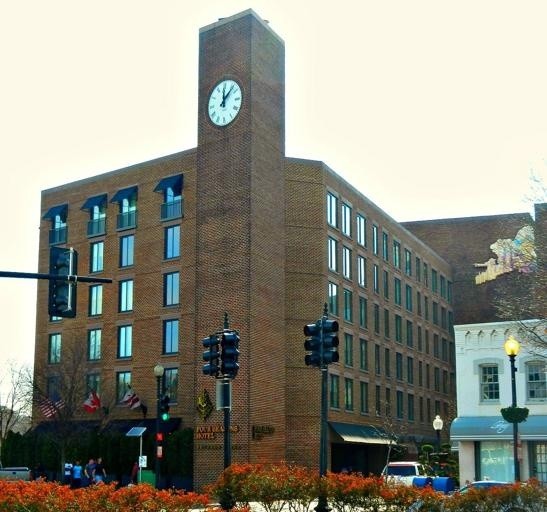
[501,335,530,482]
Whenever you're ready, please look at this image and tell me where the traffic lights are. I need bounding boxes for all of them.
[224,332,241,371]
[160,394,172,423]
[303,321,322,367]
[155,432,163,457]
[204,337,217,375]
[321,318,340,363]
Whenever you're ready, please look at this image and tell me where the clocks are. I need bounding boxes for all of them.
[208,79,242,126]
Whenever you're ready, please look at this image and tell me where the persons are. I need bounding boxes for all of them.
[69,455,107,489]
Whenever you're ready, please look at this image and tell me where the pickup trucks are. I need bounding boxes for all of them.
[0,460,34,483]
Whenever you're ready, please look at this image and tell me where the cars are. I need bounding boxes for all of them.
[378,461,435,490]
[409,481,547,511]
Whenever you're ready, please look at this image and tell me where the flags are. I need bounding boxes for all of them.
[83,389,105,414]
[37,391,67,418]
[120,388,143,409]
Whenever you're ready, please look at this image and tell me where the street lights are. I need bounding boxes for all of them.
[431,414,445,455]
[154,364,165,488]
